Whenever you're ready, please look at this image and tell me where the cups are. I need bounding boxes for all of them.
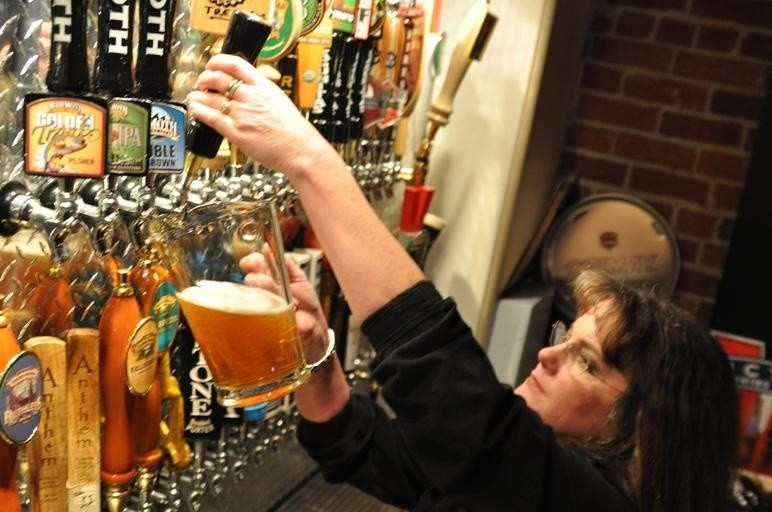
[150,199,312,407]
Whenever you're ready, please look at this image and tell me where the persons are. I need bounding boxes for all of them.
[187,53,740,512]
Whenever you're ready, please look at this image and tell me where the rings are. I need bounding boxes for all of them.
[224,79,243,100]
[222,99,233,115]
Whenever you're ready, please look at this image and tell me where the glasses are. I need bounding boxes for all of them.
[547,319,637,409]
